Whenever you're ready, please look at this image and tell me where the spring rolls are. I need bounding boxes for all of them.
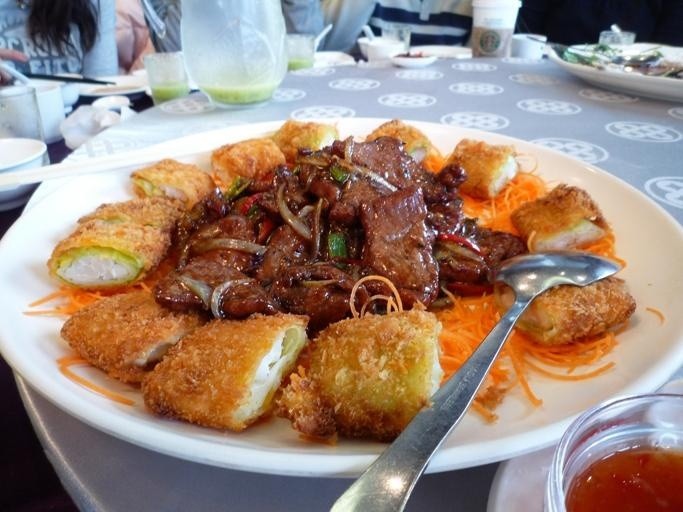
[44,116,636,442]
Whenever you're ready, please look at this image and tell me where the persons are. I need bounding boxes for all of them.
[1,0,475,86]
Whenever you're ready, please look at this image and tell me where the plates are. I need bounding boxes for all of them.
[78,74,147,95]
[541,41,683,105]
[0,115,683,481]
[388,54,439,71]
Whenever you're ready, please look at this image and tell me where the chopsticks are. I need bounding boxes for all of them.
[24,71,116,87]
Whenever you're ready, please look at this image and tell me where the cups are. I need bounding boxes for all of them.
[0,84,68,144]
[510,33,548,60]
[469,0,522,59]
[285,32,315,70]
[381,23,413,53]
[141,50,191,107]
[598,30,636,45]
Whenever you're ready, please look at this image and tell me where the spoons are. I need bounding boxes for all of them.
[328,248,624,512]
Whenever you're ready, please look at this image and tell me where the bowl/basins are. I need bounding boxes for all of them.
[0,139,47,213]
[14,73,84,113]
[356,35,407,60]
